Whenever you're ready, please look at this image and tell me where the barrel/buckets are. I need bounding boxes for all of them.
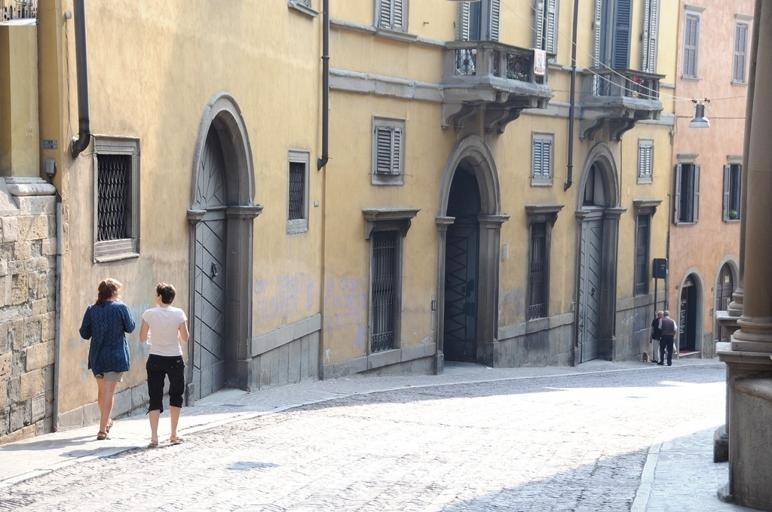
[170,436,184,444]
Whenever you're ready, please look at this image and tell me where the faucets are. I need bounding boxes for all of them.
[106,420,113,433]
[97,432,111,440]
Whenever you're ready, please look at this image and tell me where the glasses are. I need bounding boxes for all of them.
[687,97,712,130]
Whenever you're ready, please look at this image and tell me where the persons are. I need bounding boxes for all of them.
[77,276,135,442]
[650,310,664,364]
[139,280,191,447]
[658,309,679,367]
